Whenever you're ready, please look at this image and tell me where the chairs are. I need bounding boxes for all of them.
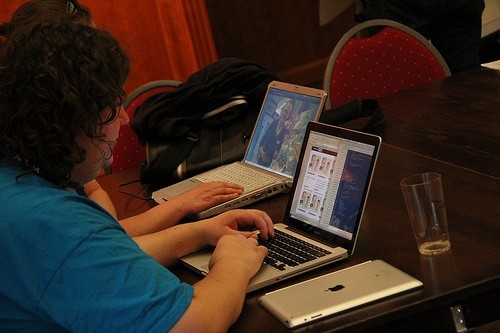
[106,81,186,171]
[323,19,452,111]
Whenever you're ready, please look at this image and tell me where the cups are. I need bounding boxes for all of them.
[399,172,451,255]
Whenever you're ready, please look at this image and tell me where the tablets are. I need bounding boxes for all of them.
[257,259,424,329]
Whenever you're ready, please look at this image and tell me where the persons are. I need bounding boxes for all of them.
[257,100,294,166]
[309,155,320,175]
[367,0,485,72]
[0,24,275,333]
[270,102,312,176]
[300,191,310,207]
[310,195,320,211]
[319,158,329,177]
[0,0,244,239]
[329,160,335,180]
[320,198,326,211]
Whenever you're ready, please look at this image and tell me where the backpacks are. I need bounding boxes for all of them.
[131,56,279,148]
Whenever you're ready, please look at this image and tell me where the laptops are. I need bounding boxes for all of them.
[152,81,328,219]
[178,120,382,294]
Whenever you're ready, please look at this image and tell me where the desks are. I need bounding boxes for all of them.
[148,63,500,333]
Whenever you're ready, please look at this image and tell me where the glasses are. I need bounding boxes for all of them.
[66,0,81,19]
